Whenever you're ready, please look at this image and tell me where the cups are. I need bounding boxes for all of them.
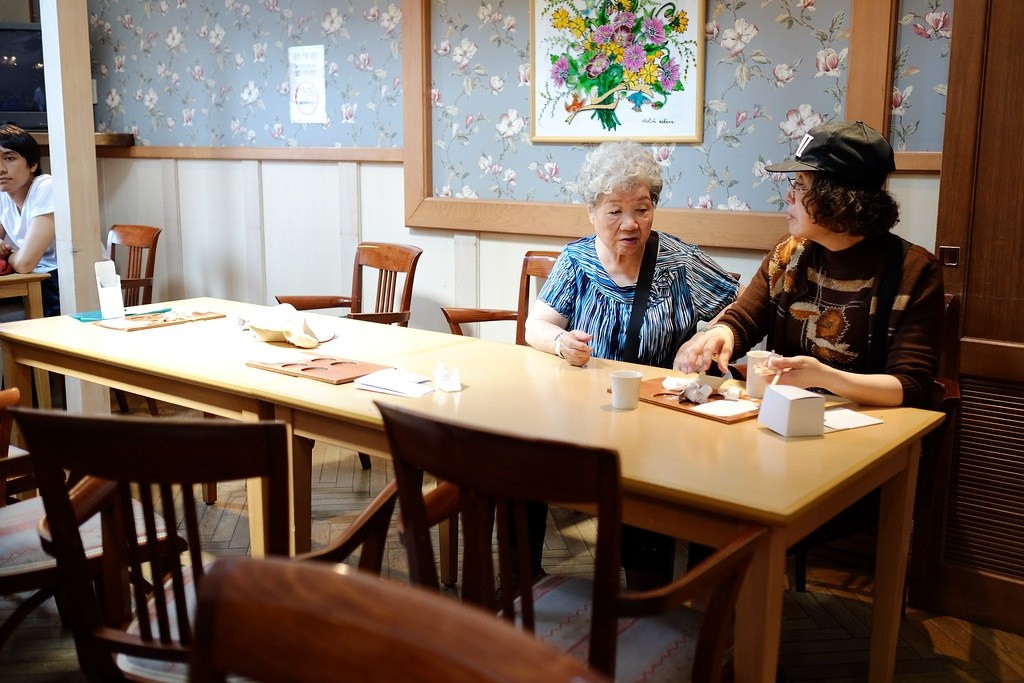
[746,350,778,399]
[610,370,642,410]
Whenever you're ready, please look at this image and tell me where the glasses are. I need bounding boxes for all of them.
[787,176,809,197]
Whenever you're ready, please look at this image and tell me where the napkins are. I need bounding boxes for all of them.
[690,398,759,417]
[823,407,884,431]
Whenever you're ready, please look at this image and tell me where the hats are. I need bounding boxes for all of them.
[764,120,897,172]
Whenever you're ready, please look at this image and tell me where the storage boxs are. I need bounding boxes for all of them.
[757,384,825,437]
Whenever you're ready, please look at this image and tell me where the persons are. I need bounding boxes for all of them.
[525,140,747,619]
[0,123,111,318]
[682,120,946,407]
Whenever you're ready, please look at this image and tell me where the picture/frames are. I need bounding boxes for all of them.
[528,0,705,144]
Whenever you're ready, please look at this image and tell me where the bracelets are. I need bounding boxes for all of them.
[552,329,567,359]
[709,324,735,362]
[698,328,710,332]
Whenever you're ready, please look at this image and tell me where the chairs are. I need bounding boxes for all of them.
[275,241,423,327]
[107,224,163,307]
[0,389,768,682]
[794,293,961,621]
[441,251,561,344]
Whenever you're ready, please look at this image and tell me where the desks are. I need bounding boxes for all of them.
[0,297,944,683]
[0,272,51,501]
